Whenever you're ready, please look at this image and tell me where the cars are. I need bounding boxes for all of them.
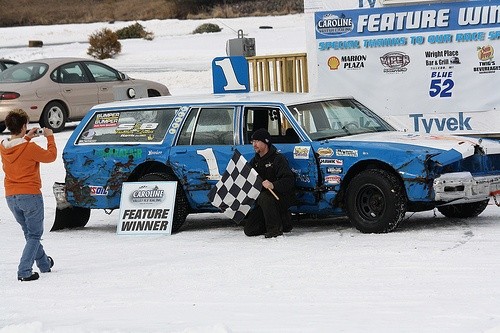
[0,58,42,80]
[0,55,172,134]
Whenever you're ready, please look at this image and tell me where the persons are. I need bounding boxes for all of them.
[0,108,58,282]
[244,127,297,239]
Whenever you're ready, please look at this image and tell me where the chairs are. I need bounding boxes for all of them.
[286,128,299,142]
[64,73,80,81]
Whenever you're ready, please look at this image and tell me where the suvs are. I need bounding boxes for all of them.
[49,52,500,236]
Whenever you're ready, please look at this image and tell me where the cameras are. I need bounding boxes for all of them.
[37,128,44,135]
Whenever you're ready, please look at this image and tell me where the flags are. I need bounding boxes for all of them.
[207,148,264,225]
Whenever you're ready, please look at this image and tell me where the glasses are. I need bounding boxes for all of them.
[251,139,260,143]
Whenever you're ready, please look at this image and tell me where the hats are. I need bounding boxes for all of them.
[251,129,271,146]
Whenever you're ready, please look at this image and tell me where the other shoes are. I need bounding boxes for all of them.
[47,256,54,272]
[18,272,39,281]
